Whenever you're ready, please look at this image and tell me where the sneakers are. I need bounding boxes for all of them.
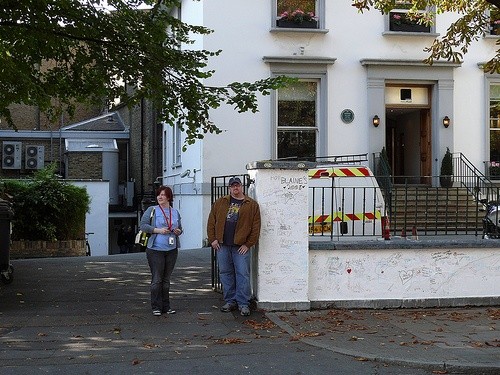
[220,302,238,312]
[239,306,251,316]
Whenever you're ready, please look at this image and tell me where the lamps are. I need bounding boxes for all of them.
[443,116,450,128]
[373,115,379,127]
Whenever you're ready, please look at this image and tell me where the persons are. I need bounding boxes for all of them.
[207,178,261,315]
[117,224,136,253]
[140,186,184,315]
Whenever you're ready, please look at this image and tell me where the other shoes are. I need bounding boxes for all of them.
[161,308,176,314]
[153,310,161,316]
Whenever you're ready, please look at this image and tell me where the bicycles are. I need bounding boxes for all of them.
[85,233,95,256]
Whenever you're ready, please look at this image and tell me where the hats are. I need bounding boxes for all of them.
[229,178,241,185]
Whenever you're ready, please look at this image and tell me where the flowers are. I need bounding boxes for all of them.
[280,9,314,24]
[490,162,500,170]
[392,15,432,25]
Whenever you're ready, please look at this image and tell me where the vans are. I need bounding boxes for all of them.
[308,166,389,239]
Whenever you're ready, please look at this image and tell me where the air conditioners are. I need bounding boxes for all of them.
[3,141,21,169]
[25,145,44,169]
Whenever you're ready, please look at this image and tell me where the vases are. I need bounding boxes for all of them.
[278,19,317,28]
[490,169,500,180]
[391,24,430,32]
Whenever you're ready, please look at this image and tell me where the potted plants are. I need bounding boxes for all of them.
[440,147,454,187]
[0,160,93,258]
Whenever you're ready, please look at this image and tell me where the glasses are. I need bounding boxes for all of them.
[229,184,241,188]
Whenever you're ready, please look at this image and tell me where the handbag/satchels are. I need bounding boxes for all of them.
[135,206,155,247]
